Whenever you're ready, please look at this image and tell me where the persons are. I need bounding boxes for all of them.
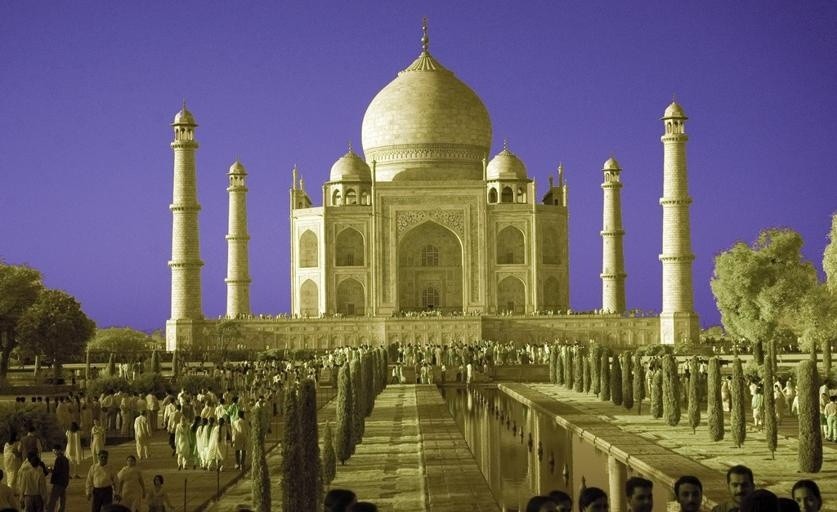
[118,359,144,382]
[91,365,98,379]
[324,488,377,510]
[192,344,387,440]
[525,465,823,511]
[0,387,251,512]
[615,339,837,442]
[390,338,596,383]
[70,370,76,387]
[216,308,660,320]
